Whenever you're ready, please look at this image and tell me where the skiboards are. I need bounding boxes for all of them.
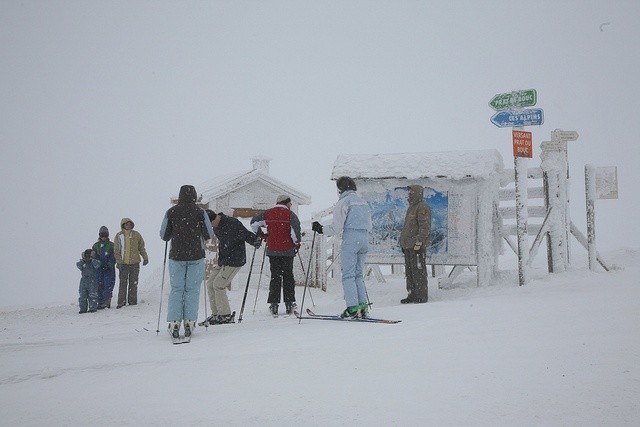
[135,310,236,332]
[269,302,298,317]
[167,324,191,344]
[293,307,402,323]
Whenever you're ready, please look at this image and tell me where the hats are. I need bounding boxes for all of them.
[85,249,92,254]
[206,210,215,220]
[99,226,109,237]
[336,176,356,194]
[277,195,291,205]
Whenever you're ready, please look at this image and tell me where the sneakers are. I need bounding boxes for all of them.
[128,300,139,307]
[359,303,372,318]
[341,305,360,319]
[100,300,110,309]
[169,320,181,338]
[79,311,86,314]
[269,303,279,315]
[97,303,103,309]
[207,314,217,322]
[116,303,125,308]
[411,299,419,303]
[400,298,413,304]
[88,310,96,312]
[209,314,231,325]
[285,302,297,314]
[184,319,194,337]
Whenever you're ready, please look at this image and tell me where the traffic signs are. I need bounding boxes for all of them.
[491,108,544,127]
[488,89,536,111]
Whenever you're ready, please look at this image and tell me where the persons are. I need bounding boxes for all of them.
[114,217,149,308]
[205,209,261,324]
[311,176,372,319]
[92,225,116,309]
[160,184,214,339]
[399,184,433,304]
[76,249,102,314]
[249,194,302,314]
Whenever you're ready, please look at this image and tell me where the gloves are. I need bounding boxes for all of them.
[90,251,96,257]
[81,252,85,259]
[312,221,323,234]
[100,264,109,271]
[116,264,123,271]
[295,242,301,253]
[143,260,148,265]
[401,247,405,254]
[413,242,423,254]
[258,231,268,242]
[254,240,261,250]
